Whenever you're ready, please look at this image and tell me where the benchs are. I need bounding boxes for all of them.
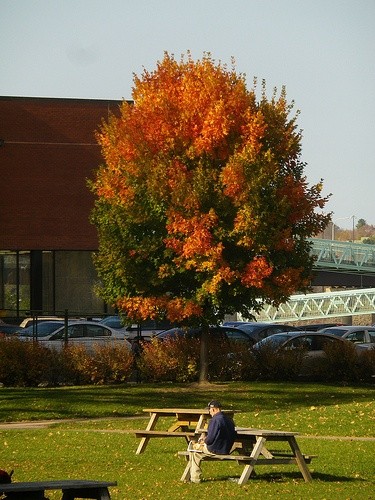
[169,422,199,431]
[260,453,318,460]
[133,430,199,441]
[177,451,253,461]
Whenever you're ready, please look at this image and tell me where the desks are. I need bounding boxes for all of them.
[0,480,117,500]
[135,408,242,455]
[180,428,314,484]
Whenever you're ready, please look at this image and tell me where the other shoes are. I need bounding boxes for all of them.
[184,475,201,484]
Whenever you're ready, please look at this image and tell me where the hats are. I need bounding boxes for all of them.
[208,401,222,408]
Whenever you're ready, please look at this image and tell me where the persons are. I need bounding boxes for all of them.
[130,336,144,359]
[188,401,235,483]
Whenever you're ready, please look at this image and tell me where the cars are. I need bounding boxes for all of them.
[0,314,375,378]
